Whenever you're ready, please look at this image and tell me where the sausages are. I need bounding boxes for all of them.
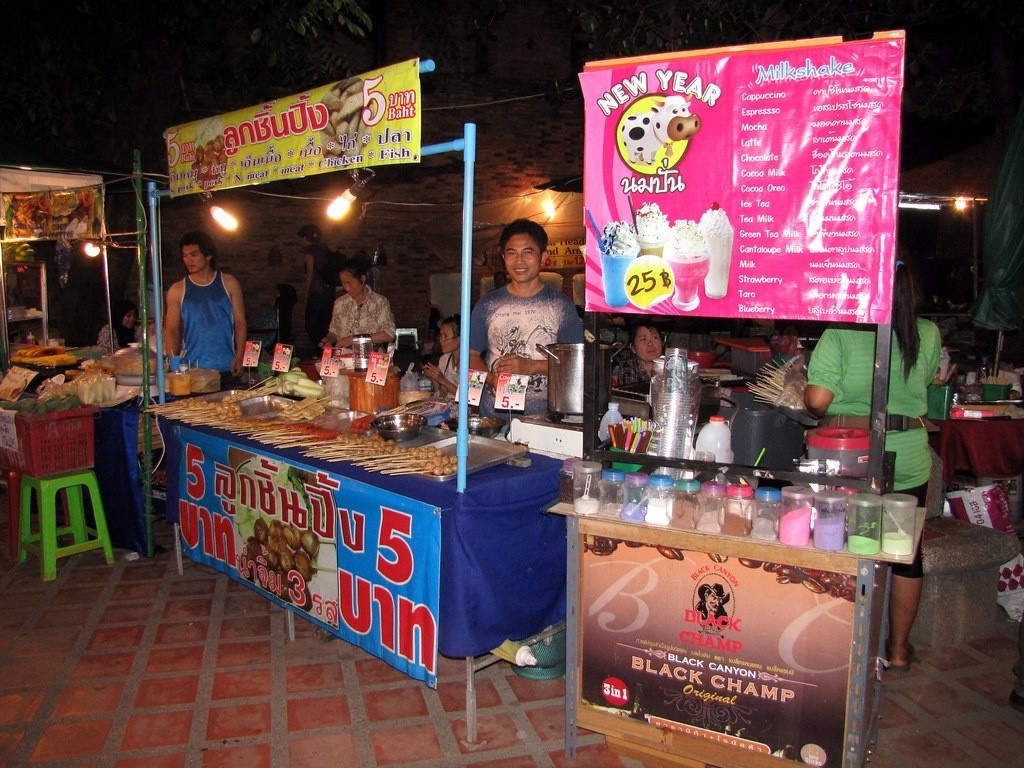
[350,414,377,434]
[286,422,342,442]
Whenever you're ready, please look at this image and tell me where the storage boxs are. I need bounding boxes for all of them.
[730,347,802,375]
[0,405,100,477]
[719,391,802,470]
[805,427,871,477]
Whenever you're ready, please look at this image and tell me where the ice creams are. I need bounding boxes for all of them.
[599,200,734,263]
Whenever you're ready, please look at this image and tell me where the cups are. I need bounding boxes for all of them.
[649,347,692,460]
[601,230,732,310]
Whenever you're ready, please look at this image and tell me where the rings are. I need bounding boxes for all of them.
[432,372,434,375]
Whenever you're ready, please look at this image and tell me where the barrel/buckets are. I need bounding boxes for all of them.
[807,428,870,477]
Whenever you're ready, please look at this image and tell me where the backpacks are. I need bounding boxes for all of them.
[312,246,348,294]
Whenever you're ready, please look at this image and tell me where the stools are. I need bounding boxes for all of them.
[0,469,115,582]
[396,324,419,349]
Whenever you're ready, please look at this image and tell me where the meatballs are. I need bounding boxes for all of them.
[177,397,243,421]
[190,134,228,190]
[335,433,459,476]
[238,520,321,611]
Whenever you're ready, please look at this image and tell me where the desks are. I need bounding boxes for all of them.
[927,419,1024,490]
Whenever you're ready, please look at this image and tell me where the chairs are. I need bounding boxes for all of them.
[429,272,586,339]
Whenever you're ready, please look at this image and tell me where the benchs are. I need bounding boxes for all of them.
[905,444,1022,649]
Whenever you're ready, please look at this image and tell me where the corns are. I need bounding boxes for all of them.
[11,345,77,366]
[274,366,325,397]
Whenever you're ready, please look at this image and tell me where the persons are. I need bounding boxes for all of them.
[165,230,246,398]
[422,314,461,401]
[805,253,942,674]
[610,318,662,388]
[318,259,396,353]
[97,300,138,353]
[469,219,585,432]
[298,224,331,357]
[1011,611,1024,713]
[967,343,1022,400]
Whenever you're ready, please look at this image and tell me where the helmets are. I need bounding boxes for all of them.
[297,224,321,241]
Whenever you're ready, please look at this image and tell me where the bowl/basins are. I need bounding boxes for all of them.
[690,353,716,367]
[371,413,428,441]
[189,368,221,392]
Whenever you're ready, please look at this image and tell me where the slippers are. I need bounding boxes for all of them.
[886,642,914,672]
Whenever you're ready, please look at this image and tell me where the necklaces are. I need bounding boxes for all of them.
[453,355,460,372]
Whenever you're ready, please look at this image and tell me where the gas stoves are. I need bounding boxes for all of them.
[505,414,583,459]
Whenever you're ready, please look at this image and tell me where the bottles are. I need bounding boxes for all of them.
[27,332,36,345]
[695,415,733,464]
[976,356,994,382]
[352,334,374,372]
[558,459,917,555]
[598,402,623,441]
[418,371,436,392]
[178,358,189,373]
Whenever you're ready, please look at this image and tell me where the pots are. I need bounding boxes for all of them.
[114,343,157,374]
[535,342,623,414]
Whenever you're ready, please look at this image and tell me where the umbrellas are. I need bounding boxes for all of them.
[970,99,1024,377]
[900,147,998,298]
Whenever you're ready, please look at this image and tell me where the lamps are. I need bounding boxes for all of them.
[373,219,387,266]
